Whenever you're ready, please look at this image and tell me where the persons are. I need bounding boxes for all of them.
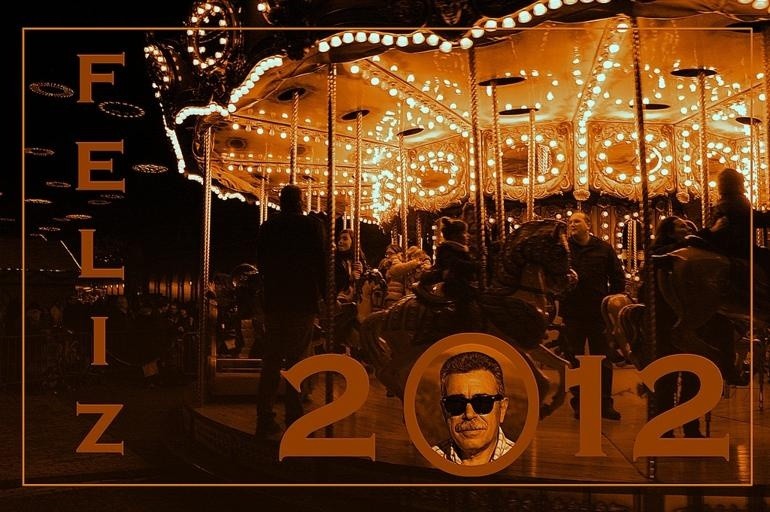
[638,214,730,438]
[557,211,627,421]
[254,183,329,436]
[209,216,770,422]
[430,350,516,467]
[706,168,770,273]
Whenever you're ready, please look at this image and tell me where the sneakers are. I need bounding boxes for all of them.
[570,387,579,410]
[603,404,621,420]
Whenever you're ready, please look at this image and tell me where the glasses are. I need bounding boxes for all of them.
[440,393,504,416]
